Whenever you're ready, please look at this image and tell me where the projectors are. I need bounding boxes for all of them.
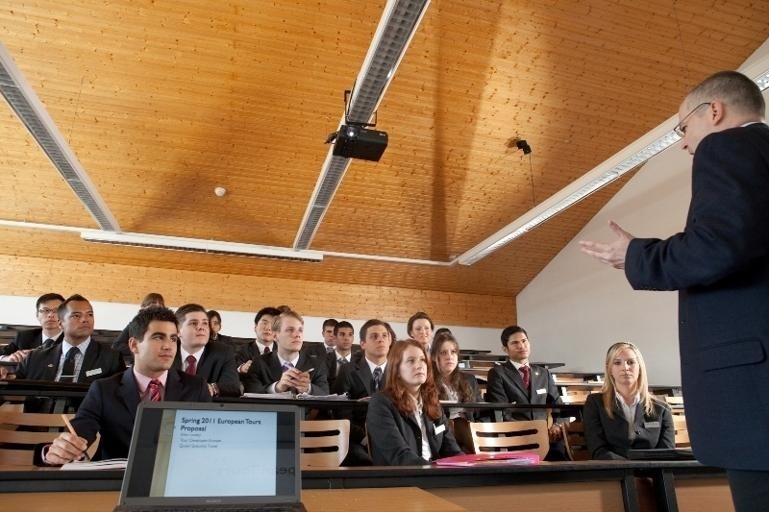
[332,125,388,162]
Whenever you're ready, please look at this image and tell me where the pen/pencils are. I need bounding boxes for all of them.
[60,413,92,462]
[290,368,314,380]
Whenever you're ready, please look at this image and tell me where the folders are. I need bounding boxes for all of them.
[432,453,539,467]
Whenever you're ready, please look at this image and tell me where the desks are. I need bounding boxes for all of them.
[461,367,606,383]
[0,459,737,512]
[1,388,689,444]
[1,359,682,398]
[0,342,566,372]
[1,323,491,355]
[459,353,509,360]
[1,486,468,512]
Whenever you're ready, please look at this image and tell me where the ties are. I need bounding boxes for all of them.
[326,346,334,354]
[263,346,272,355]
[520,365,536,392]
[146,380,163,403]
[339,358,348,366]
[44,338,54,350]
[58,347,80,383]
[183,356,197,376]
[282,363,298,397]
[372,368,384,394]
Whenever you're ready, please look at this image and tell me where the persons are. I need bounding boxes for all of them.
[233,307,280,385]
[172,303,242,398]
[337,319,389,398]
[33,305,212,467]
[305,319,339,359]
[322,322,362,388]
[407,311,434,356]
[430,334,485,423]
[244,310,330,395]
[578,71,769,511]
[112,294,165,356]
[0,292,67,366]
[10,294,126,449]
[383,322,396,347]
[365,339,464,467]
[580,340,676,511]
[485,325,571,460]
[206,309,232,345]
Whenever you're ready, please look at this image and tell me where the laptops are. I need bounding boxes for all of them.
[114,401,308,512]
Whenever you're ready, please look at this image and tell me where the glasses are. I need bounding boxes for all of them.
[37,308,59,315]
[672,101,712,138]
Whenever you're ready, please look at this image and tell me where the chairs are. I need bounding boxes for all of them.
[555,373,584,383]
[471,420,547,461]
[565,385,589,404]
[563,421,591,461]
[669,406,691,448]
[300,419,349,468]
[0,409,100,466]
[592,386,603,395]
